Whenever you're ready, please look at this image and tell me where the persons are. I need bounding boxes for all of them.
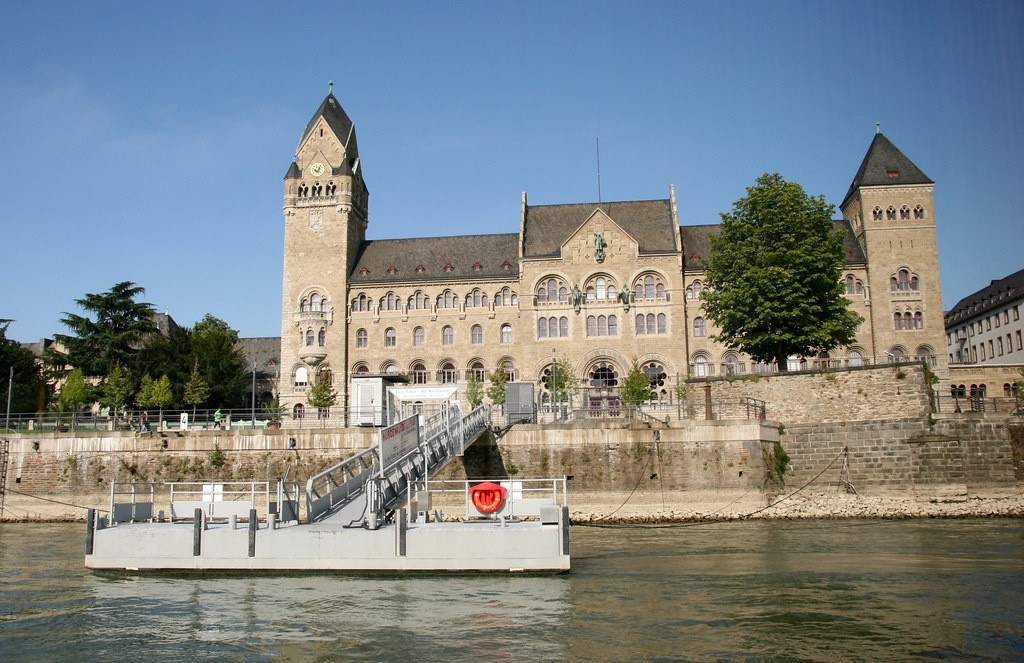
[142,411,150,431]
[213,408,222,430]
[594,233,602,253]
[571,284,581,306]
[622,284,629,304]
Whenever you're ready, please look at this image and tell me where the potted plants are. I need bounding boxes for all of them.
[261,399,288,429]
[48,394,72,432]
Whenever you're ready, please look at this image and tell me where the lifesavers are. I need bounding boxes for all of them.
[474,490,501,512]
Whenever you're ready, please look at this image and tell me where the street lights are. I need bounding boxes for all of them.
[251,357,256,429]
[552,348,558,423]
[5,366,14,434]
